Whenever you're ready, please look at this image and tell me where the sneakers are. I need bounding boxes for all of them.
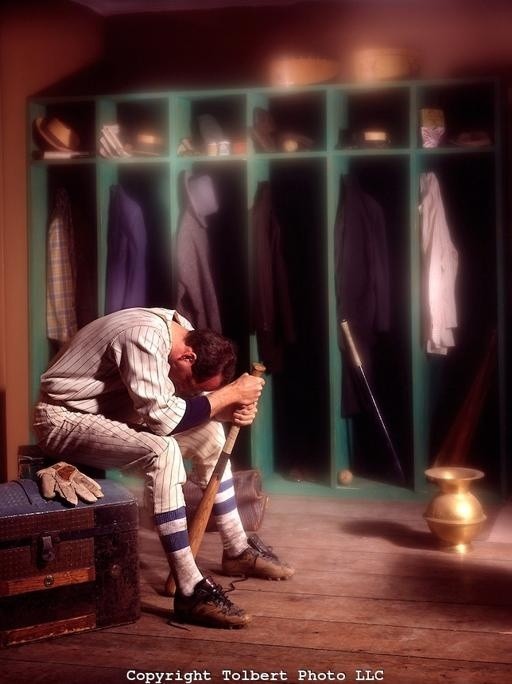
[174,576,252,630]
[222,547,295,581]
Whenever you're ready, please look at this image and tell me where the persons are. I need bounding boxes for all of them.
[33,308,295,625]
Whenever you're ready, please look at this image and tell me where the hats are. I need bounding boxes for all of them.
[182,170,221,229]
[125,131,162,156]
[34,116,82,152]
[447,130,491,147]
[355,127,390,150]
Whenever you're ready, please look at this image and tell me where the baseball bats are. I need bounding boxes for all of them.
[163,362,267,595]
[340,318,408,484]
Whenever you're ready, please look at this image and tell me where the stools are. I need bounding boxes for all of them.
[16,442,107,481]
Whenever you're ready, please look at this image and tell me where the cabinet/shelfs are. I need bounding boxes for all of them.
[26,72,511,504]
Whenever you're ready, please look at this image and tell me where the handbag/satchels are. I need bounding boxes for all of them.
[183,470,269,531]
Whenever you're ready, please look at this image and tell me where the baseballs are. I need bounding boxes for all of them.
[339,471,354,485]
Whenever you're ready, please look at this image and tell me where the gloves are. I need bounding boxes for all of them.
[37,461,104,505]
